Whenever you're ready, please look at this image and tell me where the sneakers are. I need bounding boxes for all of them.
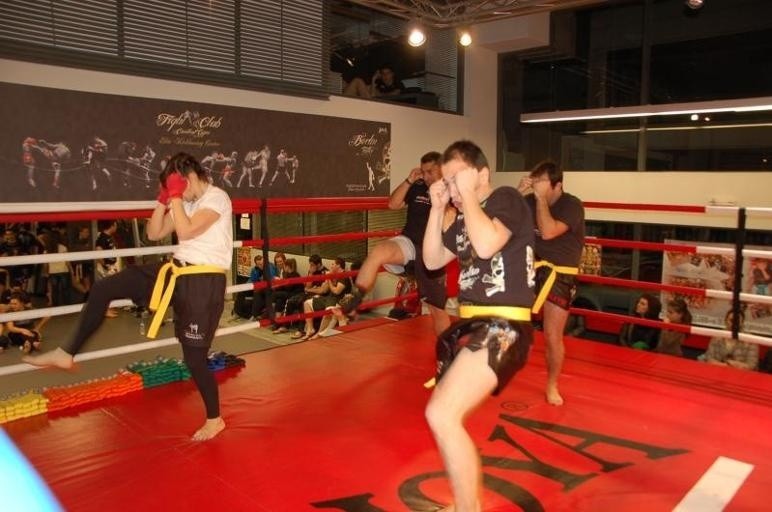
[273,328,288,334]
[291,328,315,340]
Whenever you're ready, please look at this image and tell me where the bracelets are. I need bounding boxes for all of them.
[406,178,413,187]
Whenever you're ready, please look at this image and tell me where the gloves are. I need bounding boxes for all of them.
[166,171,187,199]
[157,184,171,204]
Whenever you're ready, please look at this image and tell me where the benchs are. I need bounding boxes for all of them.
[348,312,387,321]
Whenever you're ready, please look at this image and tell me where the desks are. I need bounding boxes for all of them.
[371,93,438,106]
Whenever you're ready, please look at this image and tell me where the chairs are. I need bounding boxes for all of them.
[404,87,421,93]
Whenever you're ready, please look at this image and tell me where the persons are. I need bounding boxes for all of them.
[235,250,375,342]
[749,257,772,297]
[420,138,536,512]
[566,295,599,340]
[517,156,586,406]
[0,219,166,356]
[337,150,452,388]
[618,292,663,352]
[343,67,405,99]
[706,308,760,372]
[21,150,235,444]
[21,135,300,188]
[655,299,693,357]
